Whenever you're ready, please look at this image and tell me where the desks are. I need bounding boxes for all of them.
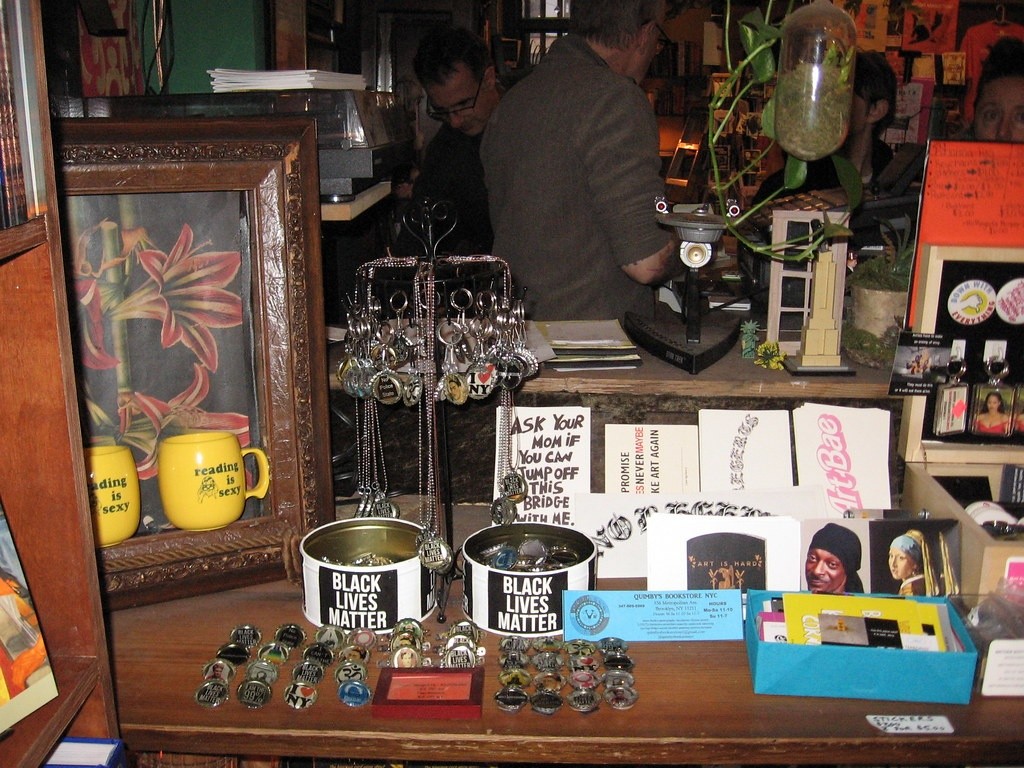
[106,495,1024,768]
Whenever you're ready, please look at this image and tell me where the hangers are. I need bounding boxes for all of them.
[992,4,1011,26]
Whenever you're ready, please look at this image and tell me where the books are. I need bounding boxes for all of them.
[209,66,392,221]
[532,317,641,370]
[641,41,721,115]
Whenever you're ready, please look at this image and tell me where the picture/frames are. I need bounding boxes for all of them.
[54,112,336,610]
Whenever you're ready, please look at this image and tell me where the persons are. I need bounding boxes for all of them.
[392,24,525,313]
[972,392,1024,435]
[950,37,1024,143]
[806,523,864,594]
[479,0,718,327]
[750,50,897,206]
[888,529,939,596]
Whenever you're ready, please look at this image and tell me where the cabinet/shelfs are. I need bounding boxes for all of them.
[0,0,126,768]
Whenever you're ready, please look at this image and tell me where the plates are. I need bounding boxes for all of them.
[964,501,1024,542]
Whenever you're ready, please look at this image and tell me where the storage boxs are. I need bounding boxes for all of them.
[898,244,1024,614]
[746,589,978,706]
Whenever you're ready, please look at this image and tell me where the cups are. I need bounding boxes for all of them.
[83,446,141,549]
[159,430,269,533]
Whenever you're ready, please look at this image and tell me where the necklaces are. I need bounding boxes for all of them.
[446,254,528,526]
[349,255,418,519]
[414,262,454,575]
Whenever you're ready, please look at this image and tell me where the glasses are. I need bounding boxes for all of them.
[426,71,484,122]
[641,20,671,56]
